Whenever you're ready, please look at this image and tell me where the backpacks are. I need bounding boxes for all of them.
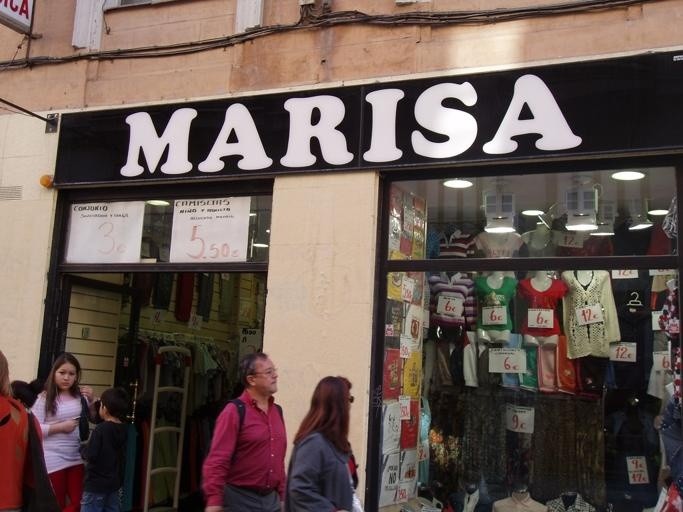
[195,399,246,458]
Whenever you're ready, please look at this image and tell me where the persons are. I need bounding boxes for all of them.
[26,353,99,511]
[0,350,62,512]
[282,376,367,511]
[201,355,287,510]
[10,378,44,409]
[427,215,624,360]
[447,486,493,511]
[602,397,661,508]
[77,387,137,511]
[492,484,548,512]
[430,481,450,506]
[545,492,597,512]
[402,481,444,511]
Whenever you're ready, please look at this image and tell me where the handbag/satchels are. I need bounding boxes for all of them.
[79,397,89,442]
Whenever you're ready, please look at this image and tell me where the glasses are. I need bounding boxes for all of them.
[254,366,278,378]
[347,395,354,404]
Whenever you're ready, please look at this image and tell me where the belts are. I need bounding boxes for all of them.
[229,483,277,497]
